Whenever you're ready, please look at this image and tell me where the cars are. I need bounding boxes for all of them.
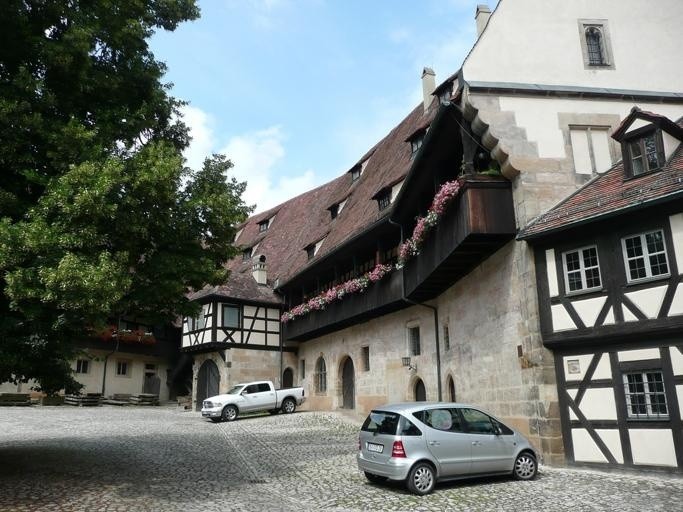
[355,401,537,494]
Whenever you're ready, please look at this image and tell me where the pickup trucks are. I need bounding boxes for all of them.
[199,380,306,423]
